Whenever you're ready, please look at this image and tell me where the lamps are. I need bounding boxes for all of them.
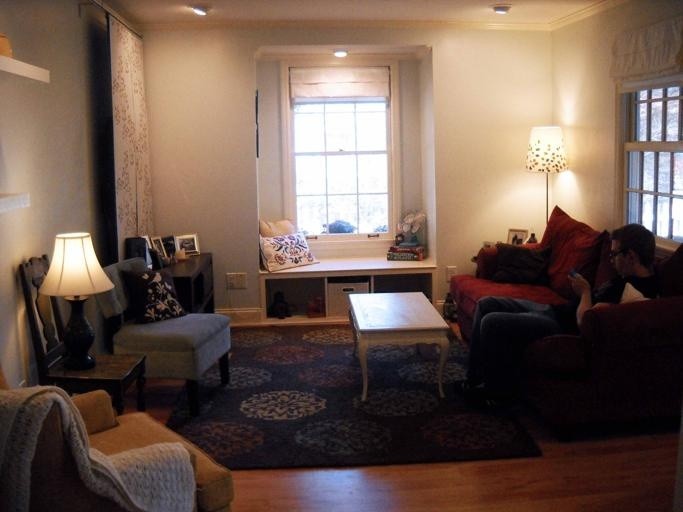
[39,232,116,369]
[444,292,453,318]
[525,124,568,225]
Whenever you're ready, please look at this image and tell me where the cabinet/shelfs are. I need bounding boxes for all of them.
[165,252,214,313]
[259,257,438,327]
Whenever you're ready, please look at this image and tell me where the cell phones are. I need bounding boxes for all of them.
[568,269,577,278]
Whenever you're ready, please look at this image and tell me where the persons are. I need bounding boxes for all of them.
[453,224,657,399]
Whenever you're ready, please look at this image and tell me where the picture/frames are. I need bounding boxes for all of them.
[507,229,528,245]
[175,232,200,256]
[141,235,152,249]
[151,237,167,257]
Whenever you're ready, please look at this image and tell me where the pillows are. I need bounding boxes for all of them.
[259,232,320,274]
[620,283,648,303]
[493,243,552,284]
[259,218,295,237]
[123,268,188,324]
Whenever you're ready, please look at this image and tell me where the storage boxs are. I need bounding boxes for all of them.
[328,282,370,317]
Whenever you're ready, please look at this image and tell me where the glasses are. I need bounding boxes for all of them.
[610,249,629,258]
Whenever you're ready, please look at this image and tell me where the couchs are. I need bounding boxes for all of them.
[445,205,683,441]
[0,369,233,512]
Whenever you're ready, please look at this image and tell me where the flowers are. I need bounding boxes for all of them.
[398,207,426,235]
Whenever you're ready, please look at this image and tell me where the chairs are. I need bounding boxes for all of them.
[103,257,232,409]
[19,252,146,414]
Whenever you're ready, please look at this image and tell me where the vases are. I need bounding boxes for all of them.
[399,232,420,246]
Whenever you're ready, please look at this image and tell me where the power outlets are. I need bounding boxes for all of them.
[226,272,247,289]
[446,266,457,282]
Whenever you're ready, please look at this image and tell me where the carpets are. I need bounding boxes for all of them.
[167,321,544,469]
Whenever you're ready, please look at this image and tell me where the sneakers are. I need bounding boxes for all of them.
[477,397,508,411]
[454,379,482,396]
